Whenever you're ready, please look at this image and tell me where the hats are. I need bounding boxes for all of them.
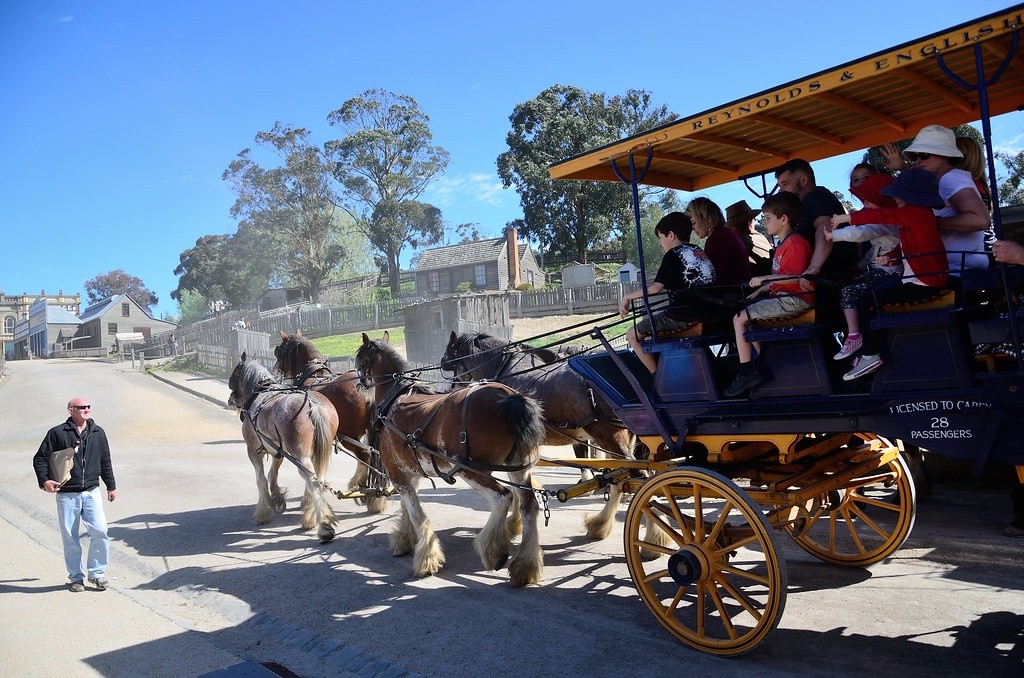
[724,200,762,227]
[902,124,964,160]
[881,169,946,210]
[848,173,900,208]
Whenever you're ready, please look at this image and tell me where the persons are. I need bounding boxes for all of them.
[619,125,1024,397]
[33,398,118,592]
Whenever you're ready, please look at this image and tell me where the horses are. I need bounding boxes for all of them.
[354,329,546,587]
[273,330,422,513]
[442,330,671,562]
[227,350,340,543]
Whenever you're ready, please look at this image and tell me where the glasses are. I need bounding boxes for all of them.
[910,151,933,161]
[72,405,90,409]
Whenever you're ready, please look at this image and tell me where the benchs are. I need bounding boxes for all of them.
[631,281,764,349]
[864,249,998,330]
[742,267,863,341]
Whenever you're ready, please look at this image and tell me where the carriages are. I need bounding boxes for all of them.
[226,0,1024,656]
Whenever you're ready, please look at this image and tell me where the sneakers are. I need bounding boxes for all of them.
[87,577,110,590]
[833,334,863,360]
[70,580,85,592]
[842,355,883,381]
[724,371,763,396]
